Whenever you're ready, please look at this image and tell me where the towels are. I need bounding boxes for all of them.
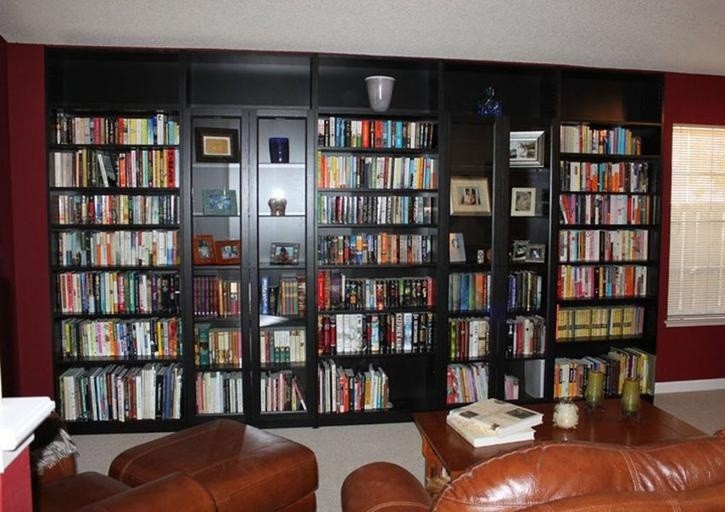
[1,394,56,450]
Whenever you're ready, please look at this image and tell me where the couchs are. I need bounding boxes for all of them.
[340,428,725,512]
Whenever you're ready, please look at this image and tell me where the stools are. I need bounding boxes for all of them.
[108,415,319,512]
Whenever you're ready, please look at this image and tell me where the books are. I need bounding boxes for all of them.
[54,113,183,419]
[554,126,654,400]
[450,397,546,437]
[318,119,435,415]
[448,271,546,402]
[191,274,308,415]
[447,416,536,448]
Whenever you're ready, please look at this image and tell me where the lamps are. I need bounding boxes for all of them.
[364,73,397,114]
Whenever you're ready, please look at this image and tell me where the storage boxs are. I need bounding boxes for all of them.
[0,432,36,512]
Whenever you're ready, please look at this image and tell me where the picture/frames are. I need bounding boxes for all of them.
[508,129,545,169]
[202,188,238,217]
[270,242,301,265]
[449,175,493,217]
[510,187,537,218]
[194,126,240,164]
[508,238,547,266]
[191,233,241,267]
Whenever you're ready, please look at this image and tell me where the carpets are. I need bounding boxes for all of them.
[653,388,725,438]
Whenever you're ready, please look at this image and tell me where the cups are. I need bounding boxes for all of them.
[269,135,289,164]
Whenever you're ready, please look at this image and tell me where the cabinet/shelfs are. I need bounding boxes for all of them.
[499,62,557,406]
[550,65,667,407]
[443,59,502,412]
[313,51,445,428]
[45,49,185,435]
[183,49,312,430]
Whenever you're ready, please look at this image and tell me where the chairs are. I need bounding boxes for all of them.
[34,470,217,512]
